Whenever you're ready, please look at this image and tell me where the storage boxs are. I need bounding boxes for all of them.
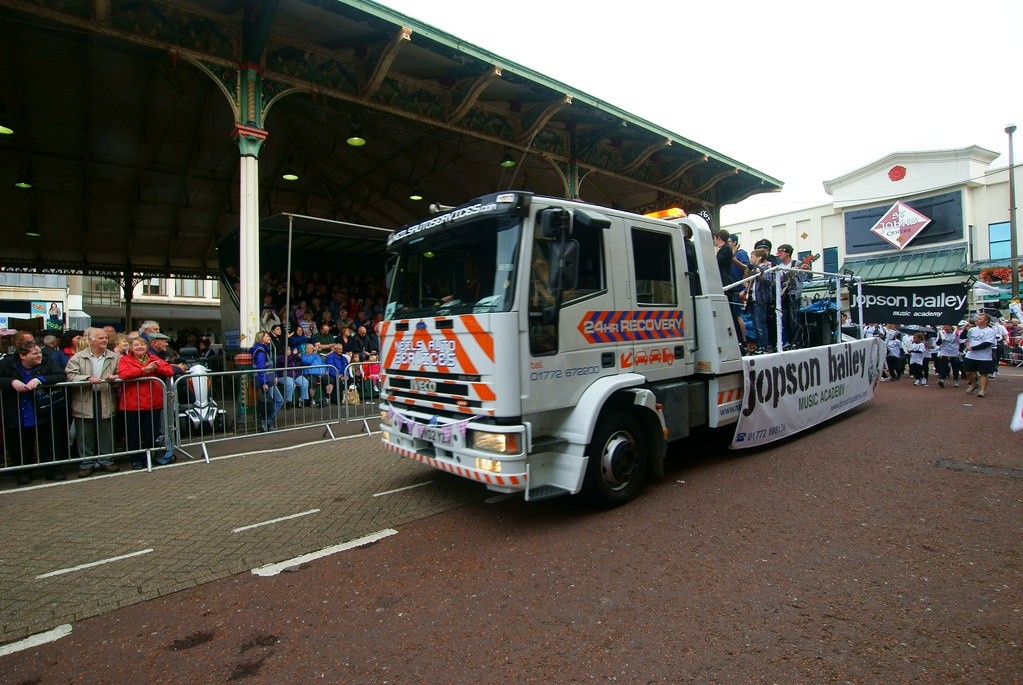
[637,280,673,304]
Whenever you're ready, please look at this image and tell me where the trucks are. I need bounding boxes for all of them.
[375,190,886,510]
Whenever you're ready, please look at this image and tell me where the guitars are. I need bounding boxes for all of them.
[771,253,820,302]
[740,254,767,313]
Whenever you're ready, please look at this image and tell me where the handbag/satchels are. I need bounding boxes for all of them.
[342,383,360,405]
[252,347,275,386]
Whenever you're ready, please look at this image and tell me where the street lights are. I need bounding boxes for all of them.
[1004,126,1020,318]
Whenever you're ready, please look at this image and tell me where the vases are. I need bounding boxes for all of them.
[988,273,1002,281]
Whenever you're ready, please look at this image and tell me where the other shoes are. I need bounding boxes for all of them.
[17,475,31,484]
[79,468,92,478]
[745,342,798,355]
[259,420,266,432]
[133,461,143,469]
[373,386,379,392]
[268,426,276,431]
[46,472,66,480]
[101,464,120,472]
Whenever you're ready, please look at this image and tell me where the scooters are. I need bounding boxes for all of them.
[172,346,228,438]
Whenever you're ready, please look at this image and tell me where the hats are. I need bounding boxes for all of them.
[754,238,772,252]
[150,333,170,339]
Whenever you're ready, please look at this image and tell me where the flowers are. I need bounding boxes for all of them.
[979,265,1012,286]
[1018,264,1023,278]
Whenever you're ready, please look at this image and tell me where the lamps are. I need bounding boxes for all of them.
[409,179,424,201]
[13,153,33,189]
[344,117,368,147]
[500,146,517,168]
[23,211,41,237]
[281,156,299,181]
[0,102,17,135]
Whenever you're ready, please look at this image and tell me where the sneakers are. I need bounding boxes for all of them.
[880,367,998,397]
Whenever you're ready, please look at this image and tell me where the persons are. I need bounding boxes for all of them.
[0,320,218,485]
[49,302,61,320]
[842,313,1023,397]
[226,266,385,432]
[681,223,813,356]
[532,247,575,307]
